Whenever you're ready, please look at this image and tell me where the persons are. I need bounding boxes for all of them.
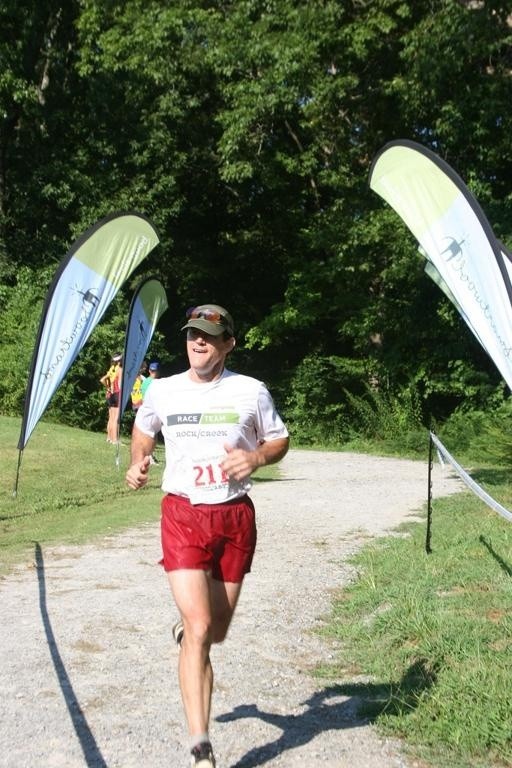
[126,304,289,768]
[100,352,160,465]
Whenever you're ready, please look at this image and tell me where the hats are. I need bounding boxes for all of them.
[180,304,234,336]
[150,363,160,371]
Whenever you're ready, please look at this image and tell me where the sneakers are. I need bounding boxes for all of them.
[174,622,183,645]
[190,742,215,768]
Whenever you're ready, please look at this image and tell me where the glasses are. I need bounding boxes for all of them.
[186,307,228,324]
